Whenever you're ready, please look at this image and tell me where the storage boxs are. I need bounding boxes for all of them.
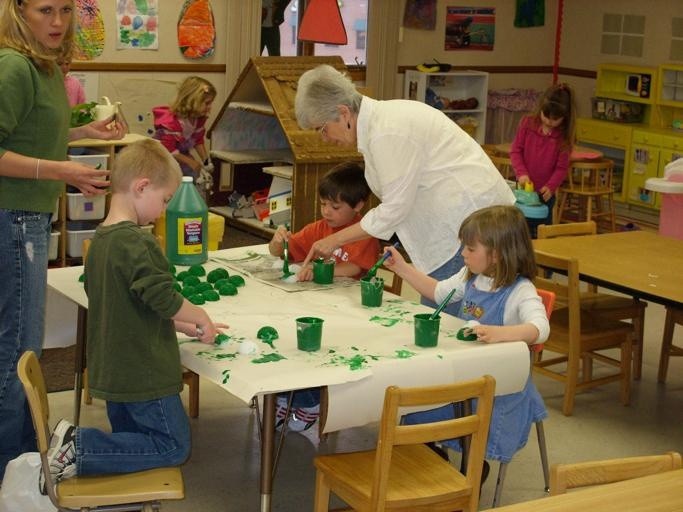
[139,224,156,236]
[48,148,111,260]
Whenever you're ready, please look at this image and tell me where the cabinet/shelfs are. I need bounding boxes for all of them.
[574,62,682,213]
[406,70,490,150]
[61,135,168,267]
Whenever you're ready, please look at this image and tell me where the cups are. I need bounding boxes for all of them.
[296,317,324,351]
[312,258,335,285]
[361,276,385,306]
[414,314,441,347]
[95,105,117,129]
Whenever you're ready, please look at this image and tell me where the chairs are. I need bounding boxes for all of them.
[16,350,185,512]
[83,238,199,418]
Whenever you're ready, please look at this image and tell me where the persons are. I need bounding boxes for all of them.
[449,16,475,47]
[260,0,294,56]
[509,83,576,246]
[262,165,384,437]
[294,66,520,320]
[150,76,213,180]
[54,53,89,112]
[380,204,550,500]
[39,136,229,497]
[0,0,127,492]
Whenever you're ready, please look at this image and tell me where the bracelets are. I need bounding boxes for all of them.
[34,157,45,183]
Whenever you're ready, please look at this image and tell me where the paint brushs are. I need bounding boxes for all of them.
[429,289,456,321]
[367,242,400,275]
[283,223,289,273]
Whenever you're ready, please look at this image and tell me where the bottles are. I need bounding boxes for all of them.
[166,175,208,266]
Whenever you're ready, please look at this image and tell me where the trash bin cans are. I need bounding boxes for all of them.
[156,211,225,251]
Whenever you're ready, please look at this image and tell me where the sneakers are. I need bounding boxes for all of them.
[275,396,288,428]
[288,404,319,432]
[38,417,77,495]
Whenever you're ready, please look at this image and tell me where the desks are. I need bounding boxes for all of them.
[48,243,530,510]
